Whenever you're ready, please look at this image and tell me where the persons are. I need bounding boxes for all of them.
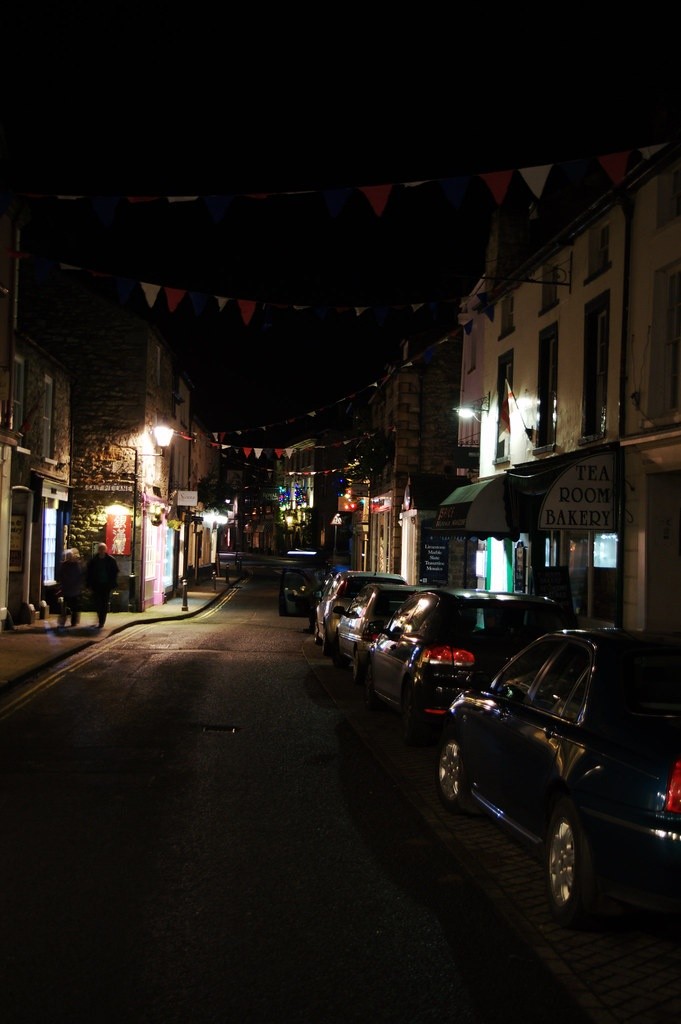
[85,543,120,628]
[55,550,83,627]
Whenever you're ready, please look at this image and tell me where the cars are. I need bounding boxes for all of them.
[357,587,579,752]
[277,562,408,655]
[332,583,439,681]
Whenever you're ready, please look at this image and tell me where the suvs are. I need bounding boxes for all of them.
[433,627,681,932]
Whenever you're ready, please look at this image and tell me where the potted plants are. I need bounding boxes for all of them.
[168,519,182,531]
[151,512,162,527]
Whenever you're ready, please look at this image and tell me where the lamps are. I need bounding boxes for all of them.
[452,391,491,418]
[138,423,175,458]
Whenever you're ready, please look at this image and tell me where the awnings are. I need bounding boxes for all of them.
[502,450,614,535]
[432,478,510,540]
